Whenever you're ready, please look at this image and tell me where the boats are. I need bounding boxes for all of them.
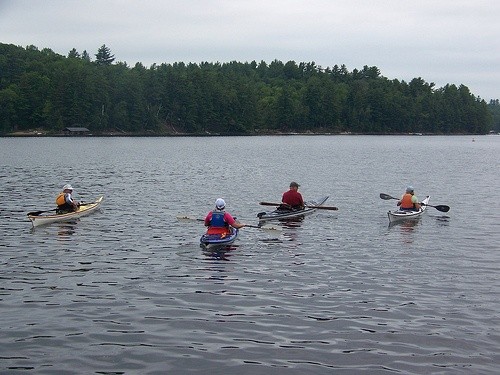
[386,195,431,229]
[257,195,329,226]
[199,216,240,251]
[26,196,105,228]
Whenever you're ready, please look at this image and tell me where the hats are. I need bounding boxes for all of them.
[290,182,301,186]
[215,199,226,209]
[62,184,75,190]
[405,186,414,192]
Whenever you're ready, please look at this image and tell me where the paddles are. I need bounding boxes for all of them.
[259,202,339,211]
[27,202,95,216]
[379,193,450,213]
[177,215,282,236]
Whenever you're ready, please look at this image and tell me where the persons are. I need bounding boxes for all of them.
[396,186,421,211]
[56,183,80,213]
[281,181,304,208]
[204,197,244,234]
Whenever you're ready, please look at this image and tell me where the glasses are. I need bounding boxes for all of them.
[69,190,72,191]
